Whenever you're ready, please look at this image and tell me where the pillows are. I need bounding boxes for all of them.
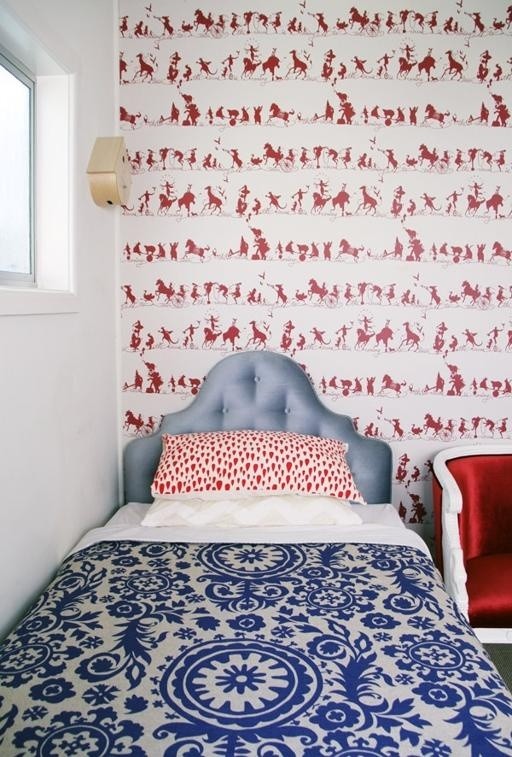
[141,499,362,528]
[150,432,367,505]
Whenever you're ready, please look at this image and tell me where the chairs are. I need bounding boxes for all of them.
[432,442,512,645]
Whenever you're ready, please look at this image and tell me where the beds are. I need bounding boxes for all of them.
[0,352,512,756]
